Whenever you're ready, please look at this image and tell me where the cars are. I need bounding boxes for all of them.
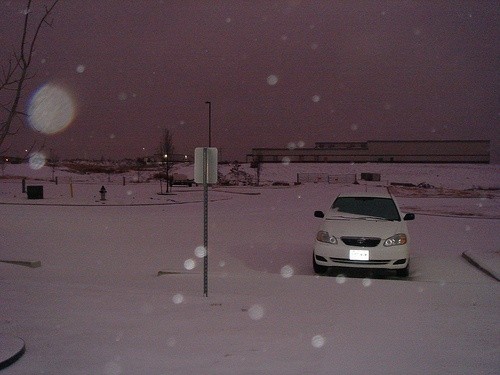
[312,190,415,276]
